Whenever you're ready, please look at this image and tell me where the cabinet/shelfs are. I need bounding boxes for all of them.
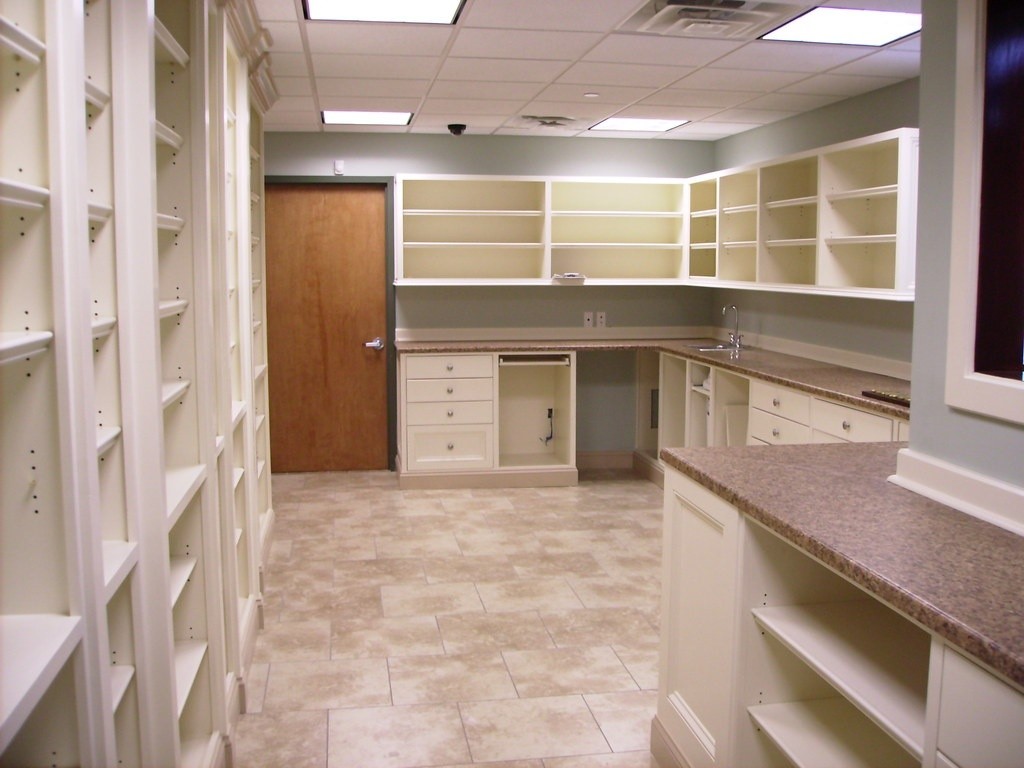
[687,126,919,303]
[0,1,273,768]
[393,172,687,286]
[658,459,739,768]
[400,353,493,472]
[659,351,687,473]
[934,636,1024,768]
[811,395,893,444]
[750,377,811,445]
[635,349,660,467]
[737,512,935,768]
[713,366,750,448]
[493,351,578,471]
[686,358,713,448]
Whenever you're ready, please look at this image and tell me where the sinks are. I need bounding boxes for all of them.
[687,343,743,352]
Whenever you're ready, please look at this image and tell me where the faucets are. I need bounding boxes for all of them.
[721,303,739,343]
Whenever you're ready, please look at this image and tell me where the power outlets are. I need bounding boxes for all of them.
[597,312,606,329]
